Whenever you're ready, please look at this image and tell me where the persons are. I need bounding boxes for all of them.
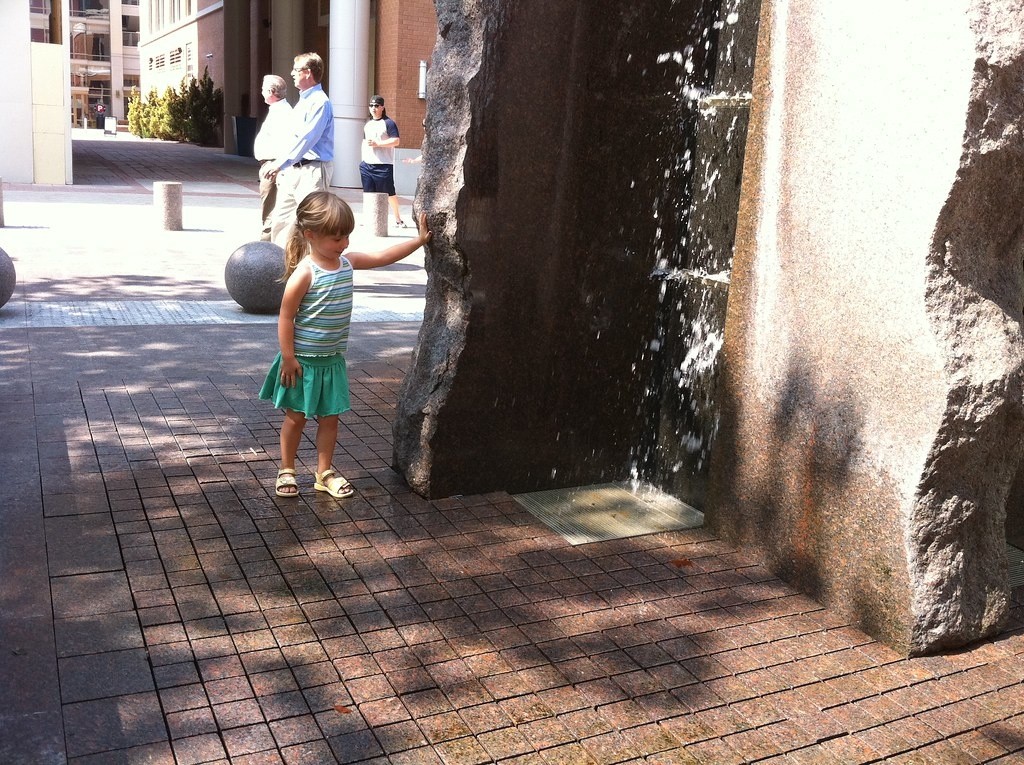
[258,191,434,498]
[264,52,335,250]
[359,94,407,228]
[253,74,294,242]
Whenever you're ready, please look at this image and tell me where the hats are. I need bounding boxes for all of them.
[370,95,384,105]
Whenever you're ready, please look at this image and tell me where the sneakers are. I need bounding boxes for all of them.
[396,221,407,228]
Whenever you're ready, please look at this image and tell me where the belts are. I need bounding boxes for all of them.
[292,159,313,168]
[258,158,276,166]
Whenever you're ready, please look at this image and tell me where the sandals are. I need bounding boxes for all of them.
[314,470,354,498]
[275,468,300,497]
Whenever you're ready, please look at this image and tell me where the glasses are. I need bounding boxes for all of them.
[369,104,379,108]
[292,68,309,72]
